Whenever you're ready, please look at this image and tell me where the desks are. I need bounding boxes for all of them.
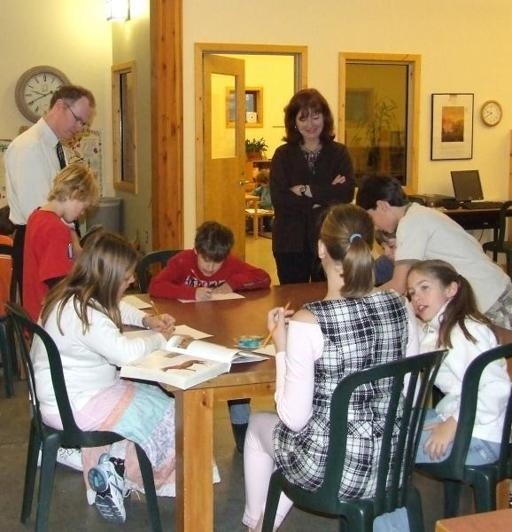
[109,280,512,531]
[440,205,512,263]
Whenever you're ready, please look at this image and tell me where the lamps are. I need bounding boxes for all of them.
[103,0,133,26]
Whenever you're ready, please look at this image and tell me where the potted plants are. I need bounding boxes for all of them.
[246,138,268,161]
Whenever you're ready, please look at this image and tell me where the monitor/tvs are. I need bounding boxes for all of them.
[451,170,483,204]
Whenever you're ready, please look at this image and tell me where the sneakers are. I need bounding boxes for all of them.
[87,452,133,524]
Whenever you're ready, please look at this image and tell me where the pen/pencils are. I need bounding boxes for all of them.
[191,269,204,287]
[150,301,168,326]
[262,301,291,348]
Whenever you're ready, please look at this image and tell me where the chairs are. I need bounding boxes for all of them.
[136,247,184,294]
[412,343,512,515]
[262,345,453,531]
[244,196,275,241]
[2,297,167,532]
[484,199,512,274]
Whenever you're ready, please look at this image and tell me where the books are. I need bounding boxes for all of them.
[120,335,269,390]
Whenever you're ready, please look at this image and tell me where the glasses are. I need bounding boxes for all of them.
[62,93,87,126]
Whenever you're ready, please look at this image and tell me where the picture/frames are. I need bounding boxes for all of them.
[107,61,139,196]
[430,92,475,160]
[335,48,422,199]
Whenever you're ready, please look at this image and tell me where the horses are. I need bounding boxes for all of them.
[159,359,208,373]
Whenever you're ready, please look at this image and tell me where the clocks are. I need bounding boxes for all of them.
[481,99,503,127]
[14,63,71,122]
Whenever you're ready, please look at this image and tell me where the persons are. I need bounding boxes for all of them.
[6,87,96,377]
[360,171,512,465]
[148,221,271,453]
[252,171,272,232]
[242,205,419,532]
[22,163,99,341]
[268,88,356,284]
[30,229,221,525]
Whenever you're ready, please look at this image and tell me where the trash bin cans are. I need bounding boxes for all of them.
[86,197,121,235]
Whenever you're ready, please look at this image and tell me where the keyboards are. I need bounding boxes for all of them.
[463,202,504,209]
